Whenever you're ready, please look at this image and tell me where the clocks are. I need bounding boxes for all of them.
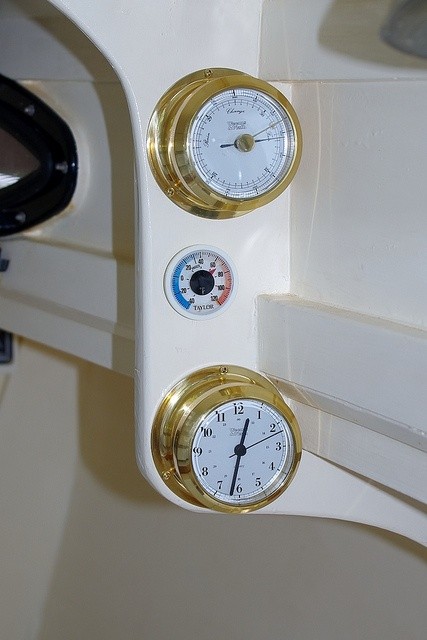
[190,397,297,507]
